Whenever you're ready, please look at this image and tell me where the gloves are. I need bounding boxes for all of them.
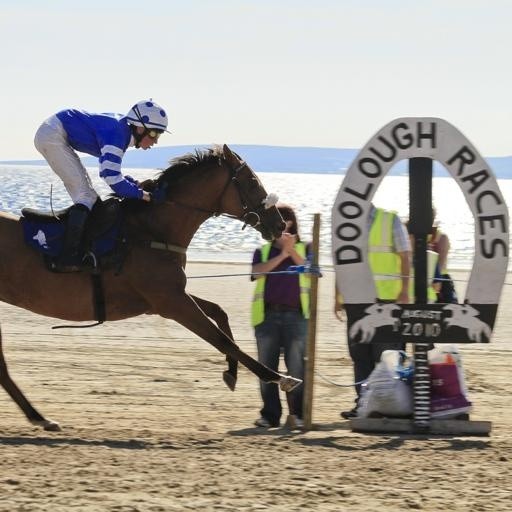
[149,187,166,205]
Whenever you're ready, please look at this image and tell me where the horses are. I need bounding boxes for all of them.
[0,142,304,433]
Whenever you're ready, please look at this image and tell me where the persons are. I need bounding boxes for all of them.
[33,97,171,275]
[401,202,450,304]
[250,204,322,430]
[333,198,412,423]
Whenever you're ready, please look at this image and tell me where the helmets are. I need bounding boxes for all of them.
[127,99,172,134]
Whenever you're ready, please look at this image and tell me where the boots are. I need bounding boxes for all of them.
[37,202,90,273]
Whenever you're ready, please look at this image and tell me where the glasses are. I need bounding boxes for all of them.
[133,104,161,141]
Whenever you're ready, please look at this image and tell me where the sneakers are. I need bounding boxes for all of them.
[340,402,383,419]
[289,414,304,430]
[255,417,272,428]
